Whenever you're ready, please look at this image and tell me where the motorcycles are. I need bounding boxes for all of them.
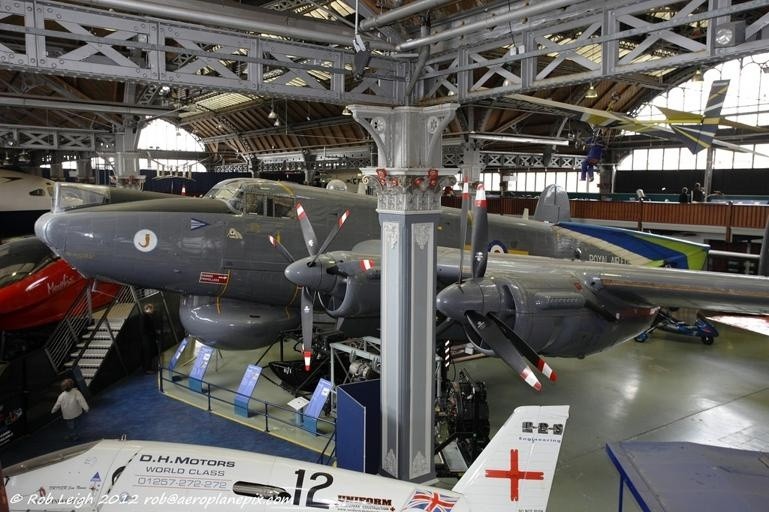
[632,307,721,345]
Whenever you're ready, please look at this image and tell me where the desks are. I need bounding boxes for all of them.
[607,440,768,511]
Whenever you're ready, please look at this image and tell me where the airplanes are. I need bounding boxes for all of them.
[29,165,769,392]
[0,232,121,330]
[0,168,92,240]
[475,78,769,158]
[1,400,579,511]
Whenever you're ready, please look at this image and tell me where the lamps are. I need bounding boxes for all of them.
[268,97,281,128]
[691,68,703,82]
[586,44,599,98]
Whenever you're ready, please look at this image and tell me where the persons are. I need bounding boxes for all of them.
[679,183,705,202]
[49,377,92,445]
[576,128,607,181]
[636,189,650,200]
